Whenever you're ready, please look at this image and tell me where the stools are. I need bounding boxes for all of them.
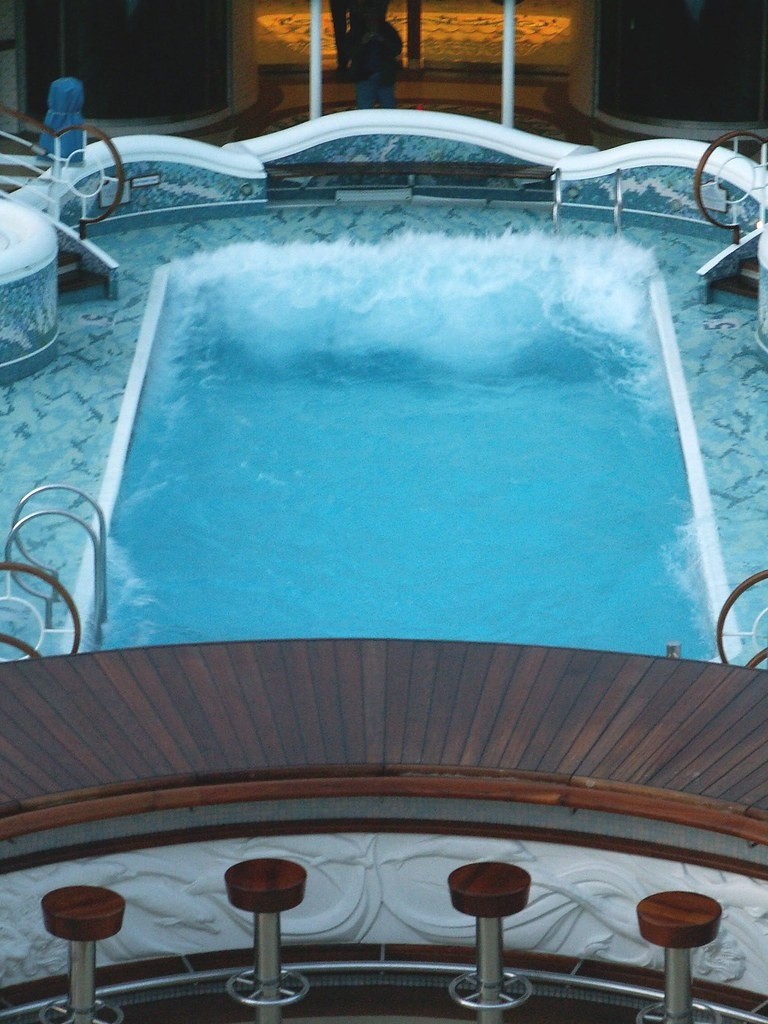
[636,891,723,1024]
[448,861,533,1024]
[39,885,127,1024]
[224,858,309,1023]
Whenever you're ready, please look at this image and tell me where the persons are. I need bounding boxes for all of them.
[328,0,403,110]
